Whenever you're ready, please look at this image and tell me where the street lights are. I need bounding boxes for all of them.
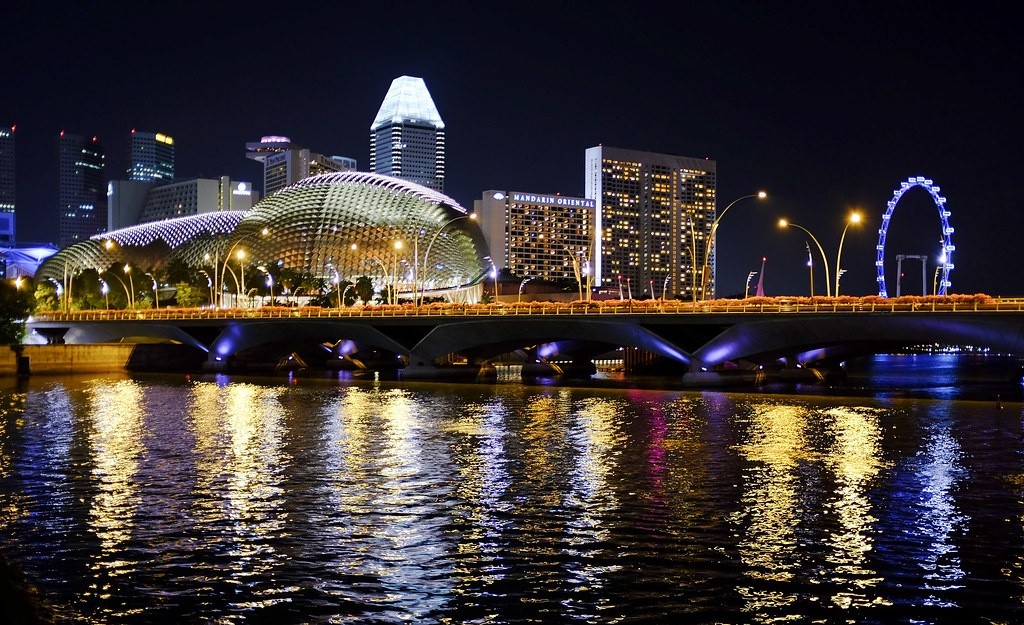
[390,241,403,303]
[420,213,478,305]
[123,264,135,310]
[834,214,863,299]
[701,191,766,300]
[349,244,391,306]
[218,229,269,307]
[204,252,240,298]
[67,239,114,309]
[778,219,830,298]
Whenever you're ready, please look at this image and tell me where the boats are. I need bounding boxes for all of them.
[1,335,172,374]
